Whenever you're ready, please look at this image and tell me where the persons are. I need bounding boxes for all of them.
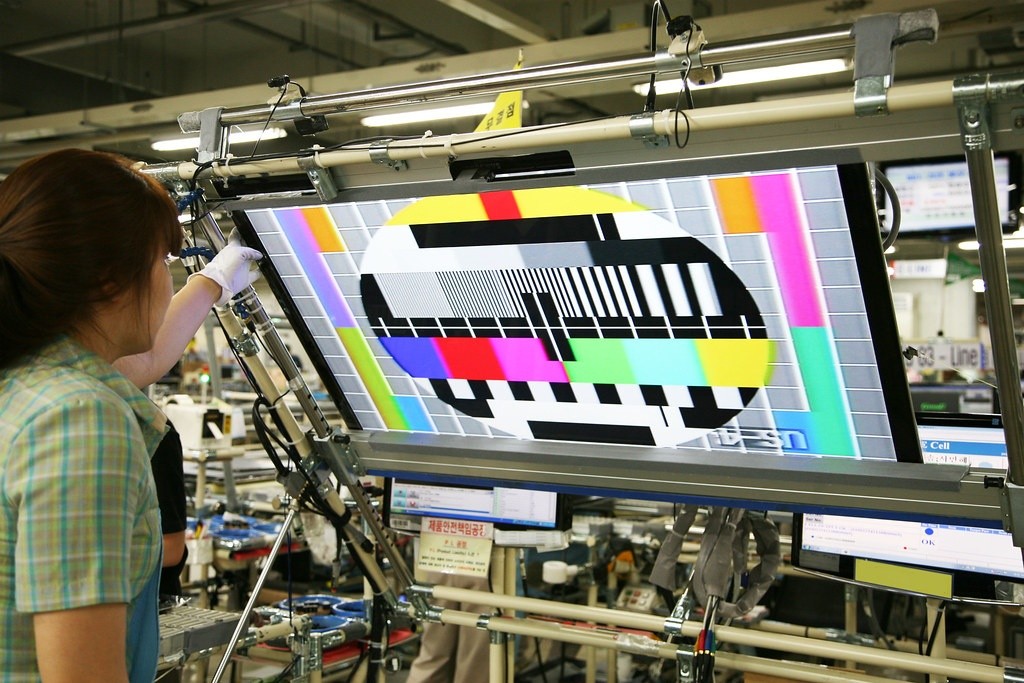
[151,420,188,597]
[0,148,263,683]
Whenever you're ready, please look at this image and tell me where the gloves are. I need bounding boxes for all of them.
[186,226,263,307]
